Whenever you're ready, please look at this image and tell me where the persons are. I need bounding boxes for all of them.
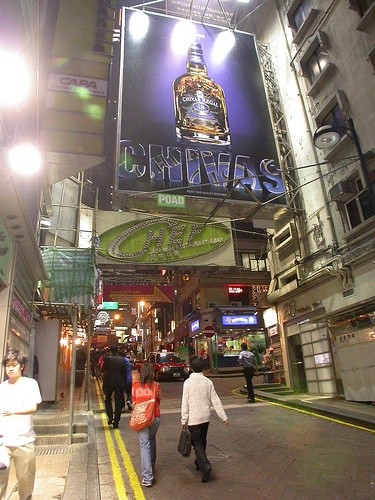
[180,359,229,482]
[131,363,160,487]
[239,342,258,403]
[0,351,42,500]
[91,344,135,429]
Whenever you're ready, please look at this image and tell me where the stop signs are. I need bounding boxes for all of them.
[204,326,214,337]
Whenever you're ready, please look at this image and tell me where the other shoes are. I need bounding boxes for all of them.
[108,417,113,425]
[113,422,119,429]
[248,399,255,403]
[126,401,132,410]
[200,464,212,483]
[246,396,251,399]
[142,480,152,486]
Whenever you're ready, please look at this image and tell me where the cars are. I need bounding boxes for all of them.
[142,352,189,382]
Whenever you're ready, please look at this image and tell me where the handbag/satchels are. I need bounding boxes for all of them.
[177,425,191,457]
[129,399,156,431]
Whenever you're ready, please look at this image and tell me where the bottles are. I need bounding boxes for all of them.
[172,44,232,146]
